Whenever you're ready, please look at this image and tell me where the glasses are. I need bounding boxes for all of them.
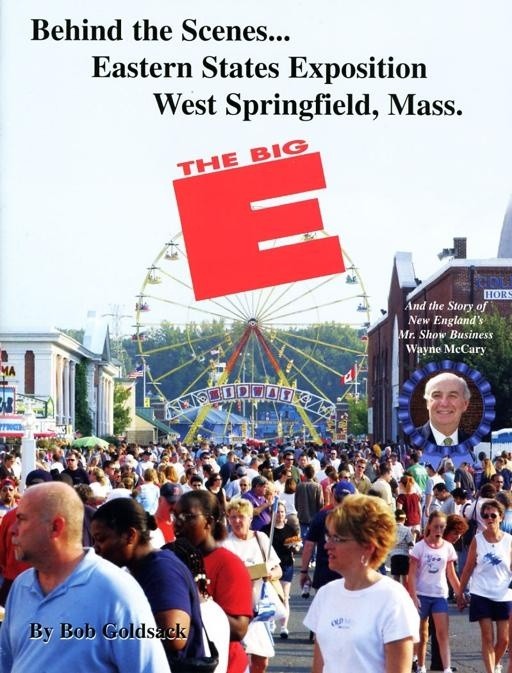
[323,534,354,544]
[169,511,205,524]
[482,512,497,519]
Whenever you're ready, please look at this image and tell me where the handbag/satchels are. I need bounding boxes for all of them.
[254,528,284,601]
[174,638,220,672]
[462,498,479,546]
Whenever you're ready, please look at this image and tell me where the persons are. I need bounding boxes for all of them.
[417,372,472,447]
[0,433,512,673]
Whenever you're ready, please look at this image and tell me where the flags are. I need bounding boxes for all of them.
[129,364,142,378]
[338,367,355,385]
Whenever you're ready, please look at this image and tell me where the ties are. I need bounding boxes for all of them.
[443,438,453,446]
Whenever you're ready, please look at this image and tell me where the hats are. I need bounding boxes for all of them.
[159,482,183,503]
[180,446,187,453]
[332,479,355,499]
[26,468,52,486]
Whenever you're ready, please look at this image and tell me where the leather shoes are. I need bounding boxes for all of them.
[430,664,456,670]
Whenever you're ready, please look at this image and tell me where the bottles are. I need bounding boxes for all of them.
[302,580,311,597]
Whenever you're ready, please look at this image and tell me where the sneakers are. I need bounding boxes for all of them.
[279,626,288,639]
[495,664,503,672]
